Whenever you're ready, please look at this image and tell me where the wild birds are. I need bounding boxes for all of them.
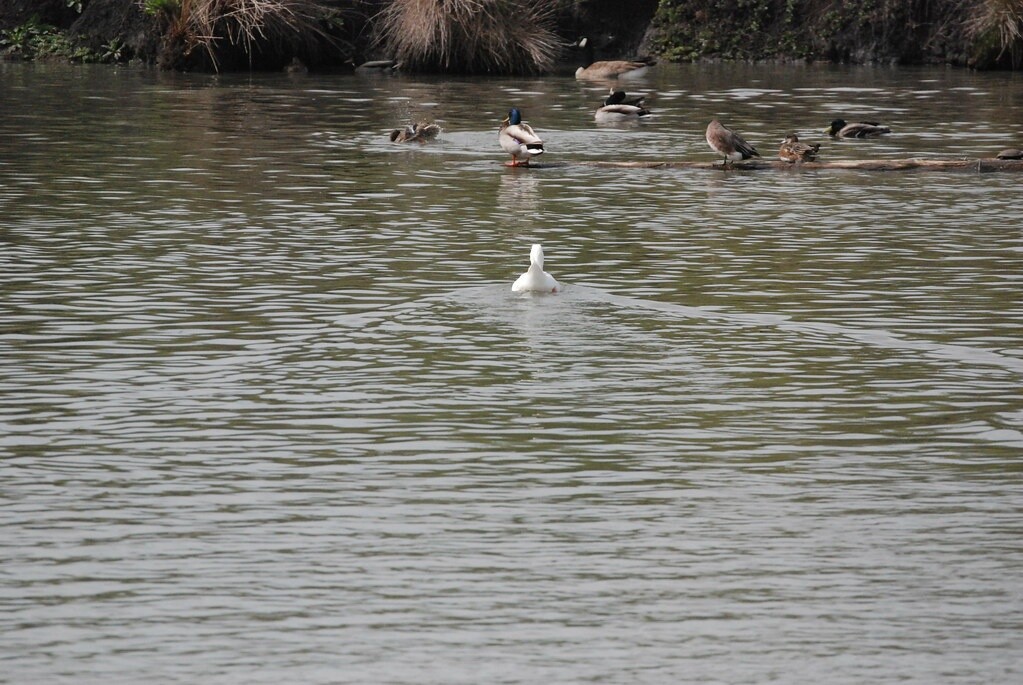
[595,88,657,119]
[823,119,892,139]
[391,122,443,145]
[779,133,821,162]
[705,118,762,164]
[575,61,657,80]
[498,108,545,166]
[512,244,560,292]
